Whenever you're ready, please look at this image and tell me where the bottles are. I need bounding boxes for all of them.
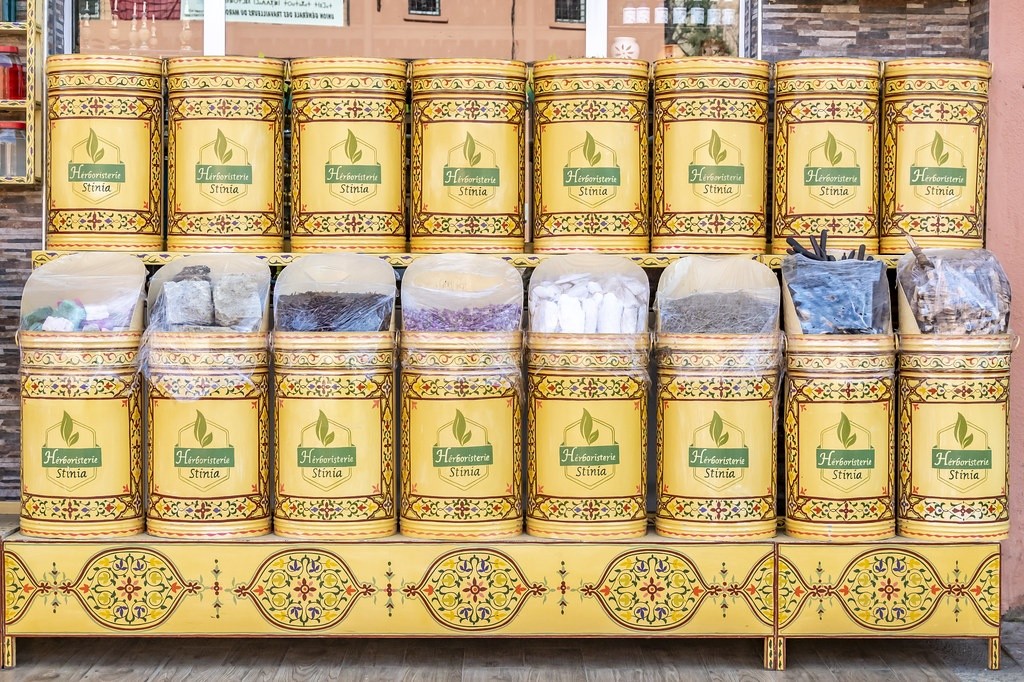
[0,46,24,100]
[0,121,26,176]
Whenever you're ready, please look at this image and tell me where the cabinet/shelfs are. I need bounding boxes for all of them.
[0,0,45,184]
[0,249,1002,670]
